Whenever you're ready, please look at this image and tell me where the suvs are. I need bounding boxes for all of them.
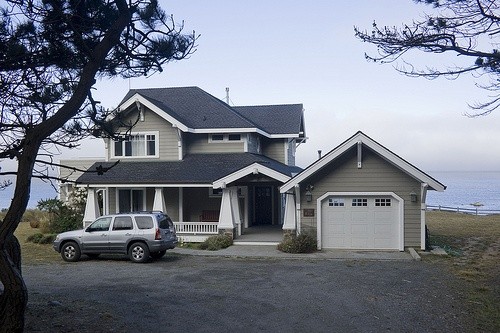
[54,211,178,264]
